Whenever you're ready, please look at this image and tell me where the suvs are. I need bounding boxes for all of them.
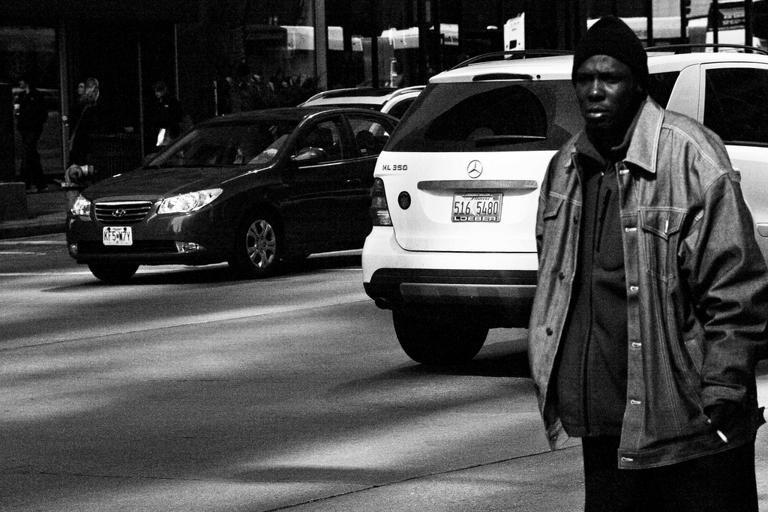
[360,43,767,367]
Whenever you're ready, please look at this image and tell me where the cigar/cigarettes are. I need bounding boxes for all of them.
[705,417,729,446]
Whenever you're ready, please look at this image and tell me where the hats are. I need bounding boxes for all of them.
[572,16,650,88]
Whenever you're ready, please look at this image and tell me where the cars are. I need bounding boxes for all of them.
[65,106,401,282]
[247,83,426,163]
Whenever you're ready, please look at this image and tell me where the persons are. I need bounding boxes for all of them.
[523,13,768,512]
[144,81,183,164]
[16,73,52,194]
[263,120,328,163]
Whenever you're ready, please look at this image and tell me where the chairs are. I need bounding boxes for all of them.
[303,126,390,160]
